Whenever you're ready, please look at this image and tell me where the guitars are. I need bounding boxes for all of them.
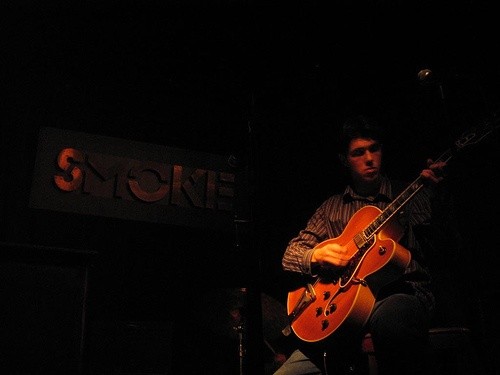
[286,116,495,347]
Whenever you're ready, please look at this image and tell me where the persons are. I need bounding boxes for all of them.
[273,116,446,375]
